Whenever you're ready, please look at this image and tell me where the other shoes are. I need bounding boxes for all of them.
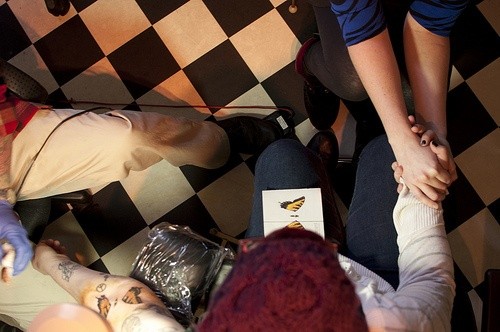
[306,131,338,164]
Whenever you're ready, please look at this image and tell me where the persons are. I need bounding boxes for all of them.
[0,84,265,332]
[23,115,456,332]
[295,0,481,210]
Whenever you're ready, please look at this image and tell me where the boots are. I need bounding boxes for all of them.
[295,34,341,130]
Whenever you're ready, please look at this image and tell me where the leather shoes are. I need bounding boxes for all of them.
[215,115,280,154]
